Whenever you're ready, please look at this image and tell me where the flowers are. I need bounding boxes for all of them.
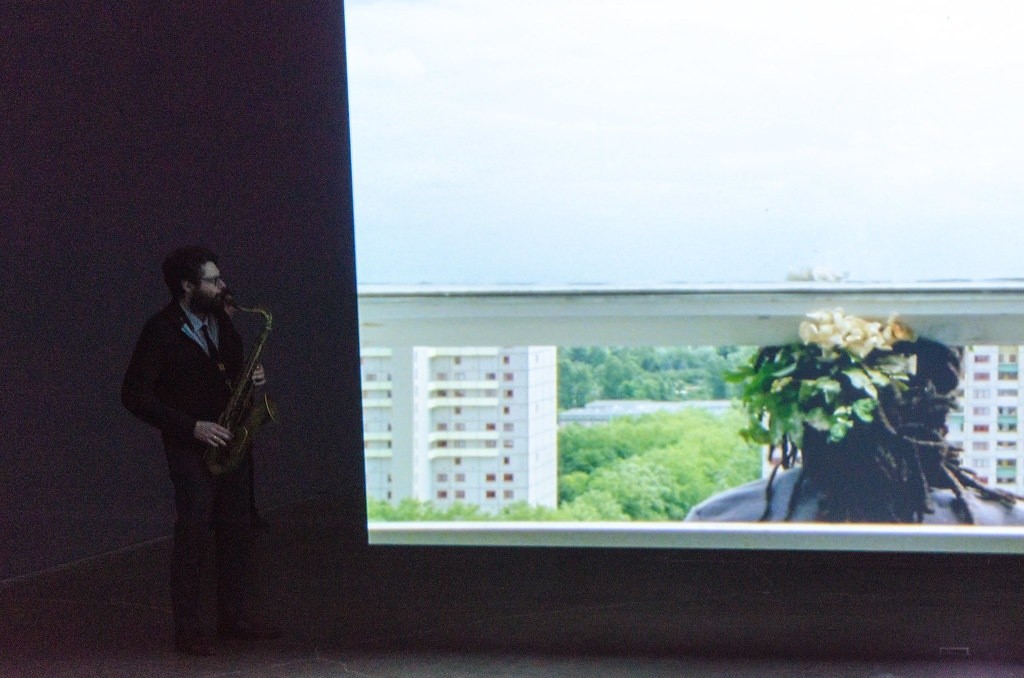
[738,313,916,450]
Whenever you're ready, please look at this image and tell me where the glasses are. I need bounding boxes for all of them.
[198,276,222,284]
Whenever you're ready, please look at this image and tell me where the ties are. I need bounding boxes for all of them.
[201,325,226,377]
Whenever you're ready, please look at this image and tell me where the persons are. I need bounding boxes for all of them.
[121,247,268,658]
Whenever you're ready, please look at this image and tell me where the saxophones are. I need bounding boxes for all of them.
[197,293,282,475]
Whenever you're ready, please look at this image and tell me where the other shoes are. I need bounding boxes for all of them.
[189,643,219,656]
[246,622,282,639]
